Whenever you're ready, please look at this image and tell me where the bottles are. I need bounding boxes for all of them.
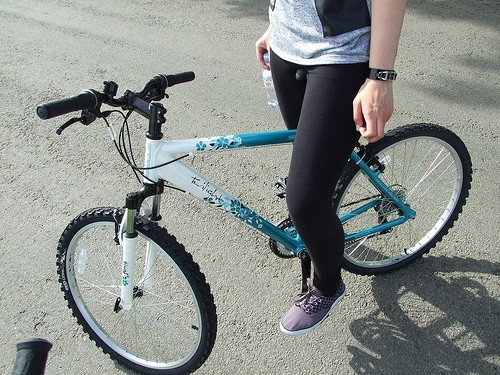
[259,50,280,110]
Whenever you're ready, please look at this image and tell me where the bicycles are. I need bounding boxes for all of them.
[37,70,472,375]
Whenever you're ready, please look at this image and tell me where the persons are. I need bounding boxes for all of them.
[254,0,407,336]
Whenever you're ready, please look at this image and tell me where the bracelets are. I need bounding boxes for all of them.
[366,68,398,82]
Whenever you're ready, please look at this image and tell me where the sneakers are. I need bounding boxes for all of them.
[280,276,347,335]
